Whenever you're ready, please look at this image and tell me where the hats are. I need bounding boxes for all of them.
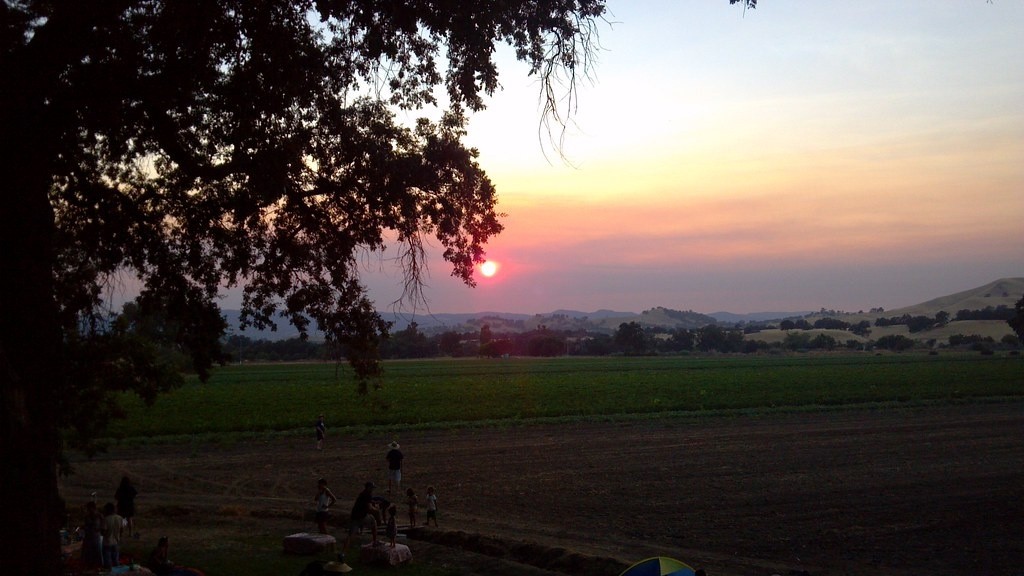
[388,440,401,450]
[363,480,378,489]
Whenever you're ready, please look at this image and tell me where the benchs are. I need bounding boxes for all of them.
[284,533,336,554]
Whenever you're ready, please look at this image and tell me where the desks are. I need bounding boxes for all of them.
[362,540,413,569]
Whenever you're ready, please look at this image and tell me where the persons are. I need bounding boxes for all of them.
[78,501,123,568]
[405,488,419,527]
[386,441,404,493]
[114,476,138,538]
[314,478,336,534]
[342,481,398,552]
[423,486,438,528]
[316,414,325,451]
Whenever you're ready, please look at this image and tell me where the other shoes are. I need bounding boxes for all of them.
[128,530,134,538]
[337,553,346,563]
[372,540,385,548]
